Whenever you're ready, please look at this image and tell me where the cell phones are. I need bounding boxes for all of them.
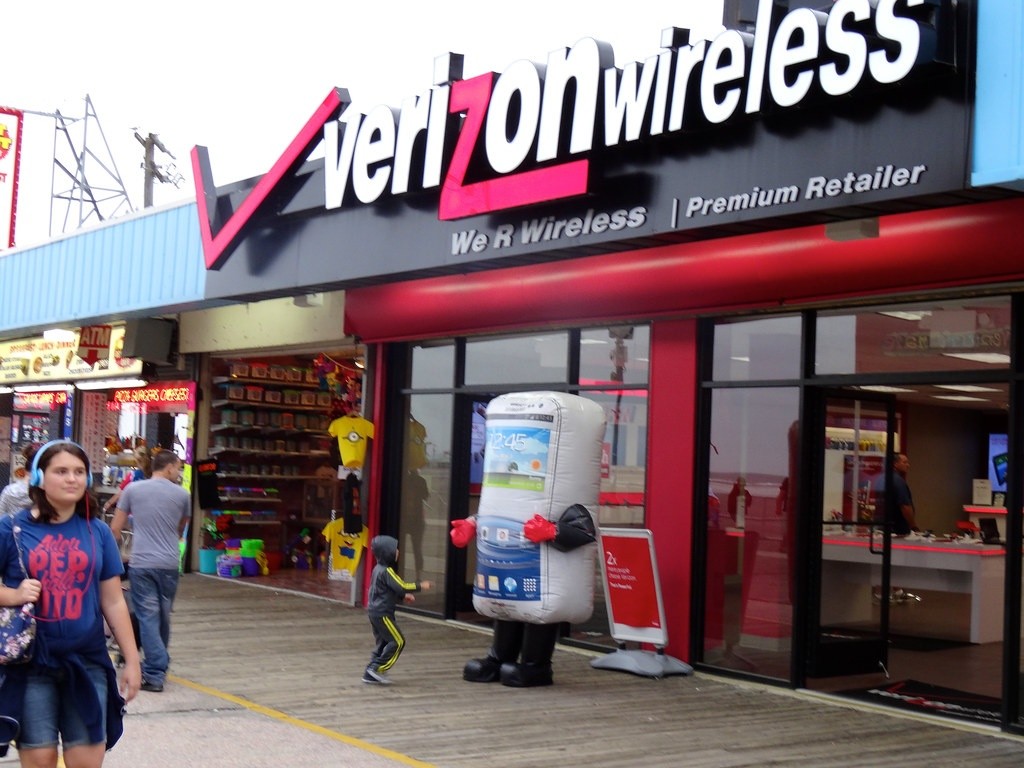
[473,396,561,611]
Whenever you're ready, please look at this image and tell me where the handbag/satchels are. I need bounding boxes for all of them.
[0,514,36,664]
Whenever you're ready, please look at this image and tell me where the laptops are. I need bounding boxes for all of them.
[979,518,1006,545]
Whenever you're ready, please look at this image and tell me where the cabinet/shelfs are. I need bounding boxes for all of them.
[207,364,351,525]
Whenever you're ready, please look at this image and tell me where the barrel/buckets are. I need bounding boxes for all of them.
[198,548,226,574]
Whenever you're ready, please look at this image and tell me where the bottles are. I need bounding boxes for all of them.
[114,662,130,705]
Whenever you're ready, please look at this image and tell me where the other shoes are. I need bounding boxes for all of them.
[139,675,164,691]
[363,676,380,684]
[367,663,396,685]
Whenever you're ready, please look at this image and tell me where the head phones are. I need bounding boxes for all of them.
[28,439,93,491]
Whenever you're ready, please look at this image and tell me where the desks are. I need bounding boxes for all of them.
[963,503,1006,542]
[822,528,1004,643]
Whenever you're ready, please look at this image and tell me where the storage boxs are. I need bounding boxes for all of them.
[210,485,279,522]
[214,362,343,434]
[300,478,343,525]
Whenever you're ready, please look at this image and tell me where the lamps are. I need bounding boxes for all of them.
[354,357,364,368]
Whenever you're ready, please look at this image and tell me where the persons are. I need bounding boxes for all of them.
[708,483,721,527]
[779,477,789,553]
[362,535,436,684]
[727,484,753,522]
[0,439,192,768]
[878,453,919,536]
